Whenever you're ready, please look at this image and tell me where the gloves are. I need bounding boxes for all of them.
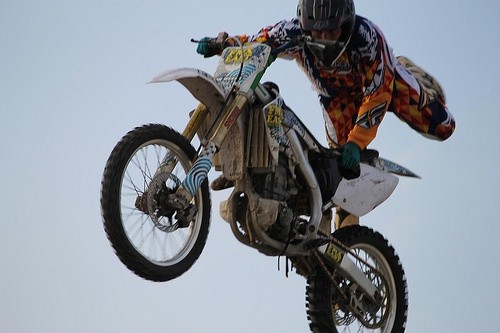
[339,141,361,170]
[197,37,214,55]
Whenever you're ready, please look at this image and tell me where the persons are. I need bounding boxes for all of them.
[196,0,457,251]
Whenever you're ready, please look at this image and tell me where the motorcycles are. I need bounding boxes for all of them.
[100,32,423,333]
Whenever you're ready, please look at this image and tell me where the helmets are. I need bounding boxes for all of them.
[296,0,356,70]
